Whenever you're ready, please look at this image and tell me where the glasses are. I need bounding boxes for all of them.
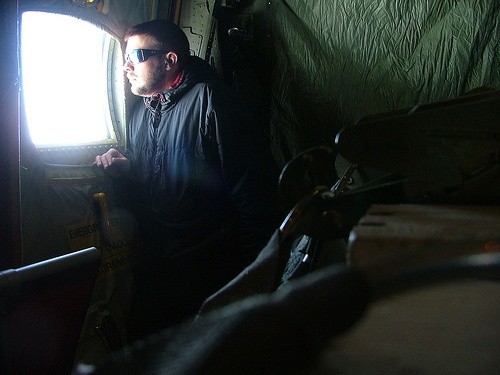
[124,48,173,64]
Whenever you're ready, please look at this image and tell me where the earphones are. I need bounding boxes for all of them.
[168,59,172,64]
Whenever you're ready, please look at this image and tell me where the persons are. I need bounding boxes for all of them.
[91,19,259,332]
